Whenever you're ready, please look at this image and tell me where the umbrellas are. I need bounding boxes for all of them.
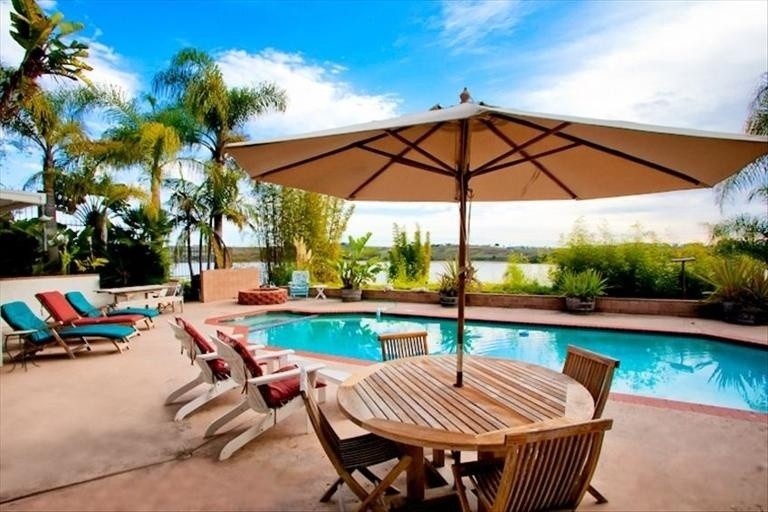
[223,88,768,388]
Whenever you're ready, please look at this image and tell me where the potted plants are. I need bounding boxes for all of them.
[434,255,461,307]
[556,266,616,313]
[697,253,768,325]
[333,232,388,302]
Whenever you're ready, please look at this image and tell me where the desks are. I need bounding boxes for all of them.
[95,284,180,304]
[313,285,328,300]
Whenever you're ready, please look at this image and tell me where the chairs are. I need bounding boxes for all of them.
[0,290,159,373]
[287,271,310,300]
[162,315,326,462]
